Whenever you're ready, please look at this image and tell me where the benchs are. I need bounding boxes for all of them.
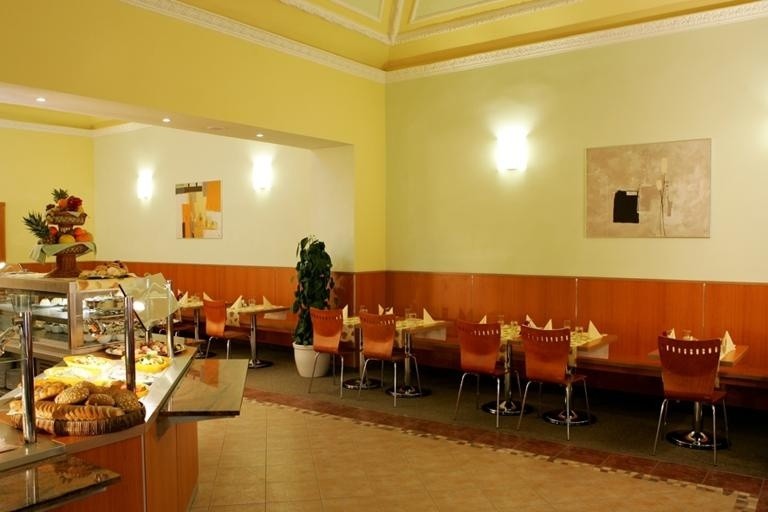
[350,306,768,395]
[174,289,303,349]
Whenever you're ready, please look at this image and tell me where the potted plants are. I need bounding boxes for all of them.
[292,235,335,379]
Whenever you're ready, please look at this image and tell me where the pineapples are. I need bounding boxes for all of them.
[21,212,57,243]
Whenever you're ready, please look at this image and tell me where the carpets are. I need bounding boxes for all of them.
[188,377,768,512]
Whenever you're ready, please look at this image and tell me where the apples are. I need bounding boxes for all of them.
[50,227,57,236]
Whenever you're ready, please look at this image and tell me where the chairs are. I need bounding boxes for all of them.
[651,336,730,466]
[357,311,423,408]
[306,307,367,398]
[516,325,589,441]
[453,319,524,429]
[197,299,251,360]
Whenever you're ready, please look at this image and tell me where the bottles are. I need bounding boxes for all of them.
[6,369,22,390]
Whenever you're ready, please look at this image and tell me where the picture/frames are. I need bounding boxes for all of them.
[583,137,712,238]
[168,178,223,239]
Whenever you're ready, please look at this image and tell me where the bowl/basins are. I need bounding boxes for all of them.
[83,333,125,344]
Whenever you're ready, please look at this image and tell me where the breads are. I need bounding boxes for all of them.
[9,380,141,421]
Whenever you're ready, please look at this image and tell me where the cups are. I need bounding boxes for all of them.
[249,295,256,309]
[563,320,571,329]
[575,326,583,343]
[408,313,416,329]
[496,315,504,327]
[681,330,691,341]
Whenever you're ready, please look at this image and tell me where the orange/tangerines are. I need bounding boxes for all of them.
[74,227,91,241]
[58,198,67,208]
